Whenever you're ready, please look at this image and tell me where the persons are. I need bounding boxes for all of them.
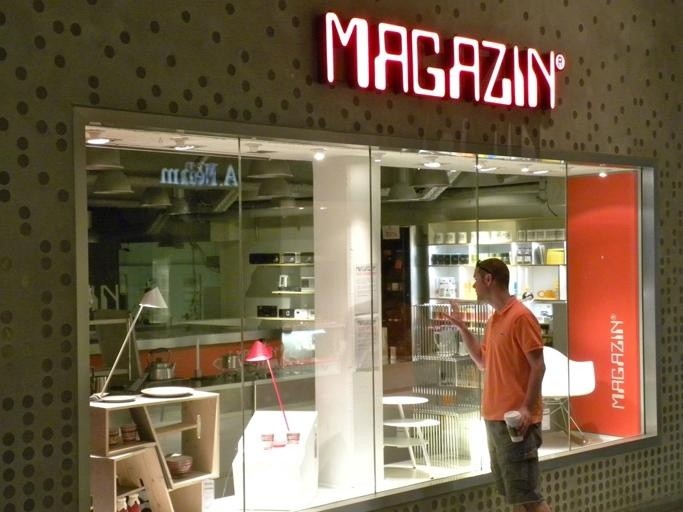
[438,257,553,511]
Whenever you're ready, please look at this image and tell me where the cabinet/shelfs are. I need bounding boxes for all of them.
[90,389,221,510]
[222,252,316,323]
[383,395,439,480]
[90,313,133,383]
[382,224,423,363]
[426,216,568,358]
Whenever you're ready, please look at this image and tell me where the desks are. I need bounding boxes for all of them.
[141,364,314,422]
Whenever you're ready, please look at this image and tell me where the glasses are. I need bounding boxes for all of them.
[477,260,495,279]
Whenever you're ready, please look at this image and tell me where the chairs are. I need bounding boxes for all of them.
[540,346,598,447]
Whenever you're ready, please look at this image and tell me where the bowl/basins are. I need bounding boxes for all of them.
[166,455,193,476]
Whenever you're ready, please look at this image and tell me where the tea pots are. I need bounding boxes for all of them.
[144,347,177,382]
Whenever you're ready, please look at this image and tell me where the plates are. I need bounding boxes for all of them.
[100,395,137,403]
[140,385,195,398]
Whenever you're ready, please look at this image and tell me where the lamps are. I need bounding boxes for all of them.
[245,341,300,448]
[87,146,296,216]
[383,166,450,203]
[89,286,168,405]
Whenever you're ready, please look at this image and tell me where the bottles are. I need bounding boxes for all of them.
[438,276,457,298]
[116,493,141,512]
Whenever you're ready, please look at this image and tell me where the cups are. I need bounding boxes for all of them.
[430,230,512,264]
[431,324,460,358]
[457,340,471,357]
[503,410,526,444]
[120,423,137,445]
[108,426,120,449]
[537,290,556,298]
